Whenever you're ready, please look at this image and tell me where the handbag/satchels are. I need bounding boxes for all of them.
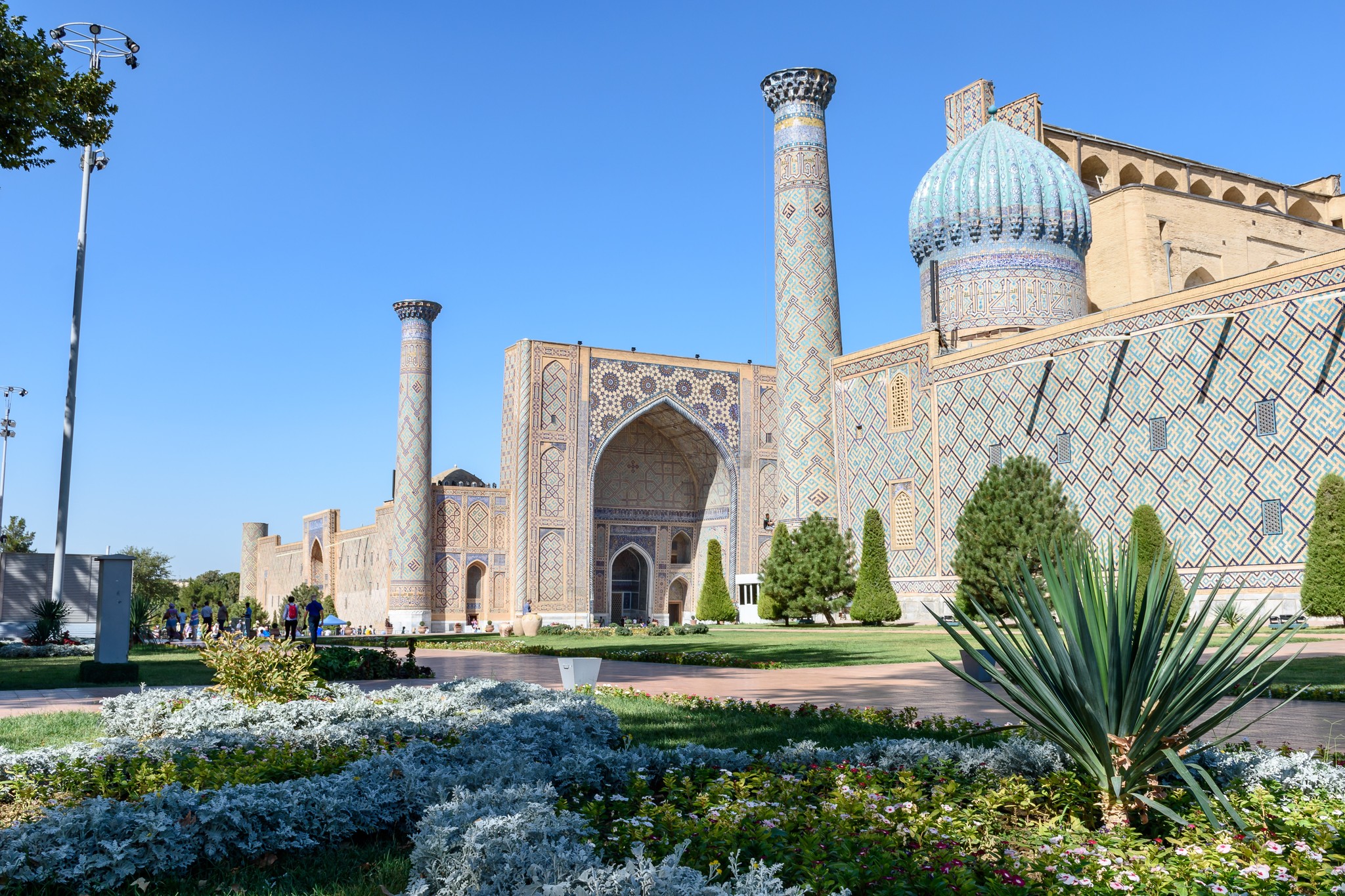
[165,609,169,619]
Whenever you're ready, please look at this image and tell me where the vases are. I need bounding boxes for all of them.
[499,622,512,637]
[453,626,462,633]
[652,622,658,626]
[522,612,543,637]
[385,627,394,634]
[512,615,523,636]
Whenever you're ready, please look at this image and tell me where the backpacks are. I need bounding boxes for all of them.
[288,604,297,619]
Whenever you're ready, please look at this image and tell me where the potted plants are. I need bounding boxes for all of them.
[690,615,697,625]
[624,619,634,626]
[591,620,601,628]
[344,621,353,635]
[270,623,279,637]
[486,621,494,632]
[417,621,427,633]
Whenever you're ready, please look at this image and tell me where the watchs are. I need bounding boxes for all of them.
[304,620,306,621]
[281,621,284,622]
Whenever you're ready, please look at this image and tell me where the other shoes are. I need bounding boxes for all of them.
[192,638,196,639]
[214,636,218,638]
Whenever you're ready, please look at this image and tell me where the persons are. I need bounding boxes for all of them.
[214,601,228,639]
[148,618,247,640]
[241,602,252,629]
[281,596,298,643]
[523,599,532,614]
[190,603,200,641]
[202,600,213,637]
[178,607,188,640]
[303,595,324,646]
[252,620,323,636]
[324,624,376,635]
[162,604,180,638]
[401,626,429,634]
[598,614,651,627]
[469,617,482,633]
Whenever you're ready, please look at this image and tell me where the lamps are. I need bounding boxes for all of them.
[939,615,959,622]
[796,616,813,620]
[1269,614,1306,625]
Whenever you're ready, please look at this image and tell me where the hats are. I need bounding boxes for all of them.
[215,623,219,626]
[245,602,250,605]
[198,624,202,627]
[301,624,307,632]
[256,620,260,623]
[216,601,222,604]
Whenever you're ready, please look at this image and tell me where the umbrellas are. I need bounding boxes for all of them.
[319,613,347,625]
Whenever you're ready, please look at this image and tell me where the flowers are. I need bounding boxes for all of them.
[454,622,461,627]
[652,618,659,623]
[384,616,393,627]
